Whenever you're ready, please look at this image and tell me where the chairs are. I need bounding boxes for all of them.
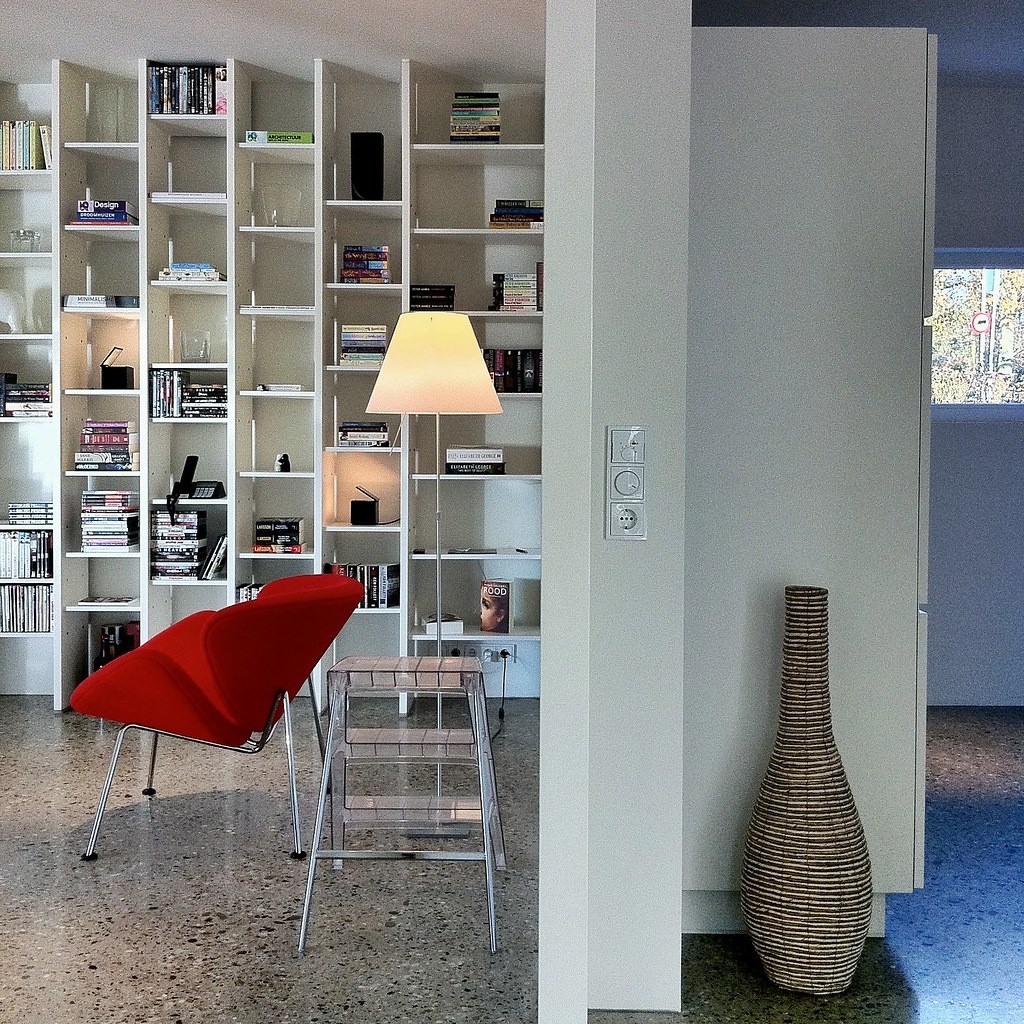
[70,574,364,864]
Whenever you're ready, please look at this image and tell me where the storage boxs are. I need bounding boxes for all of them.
[100,347,134,389]
[350,485,380,526]
[420,611,464,635]
[245,130,314,143]
[446,445,505,463]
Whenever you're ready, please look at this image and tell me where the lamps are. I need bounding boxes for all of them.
[364,311,504,838]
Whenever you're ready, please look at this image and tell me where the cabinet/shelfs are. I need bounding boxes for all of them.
[0,57,545,699]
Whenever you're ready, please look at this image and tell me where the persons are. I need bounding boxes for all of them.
[481,583,507,631]
[217,71,222,81]
[222,69,227,81]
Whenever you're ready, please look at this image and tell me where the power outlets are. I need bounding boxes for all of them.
[447,644,465,657]
[497,644,515,663]
[465,644,481,658]
[606,499,647,541]
[429,643,447,657]
[480,645,497,663]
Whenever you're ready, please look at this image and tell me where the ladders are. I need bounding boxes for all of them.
[299,655,507,955]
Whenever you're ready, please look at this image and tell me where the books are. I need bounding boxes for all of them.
[0,372,53,418]
[235,583,265,603]
[448,92,502,144]
[150,368,228,418]
[245,130,314,144]
[257,383,302,391]
[252,516,308,554]
[482,348,542,393]
[340,324,388,369]
[145,63,229,115]
[445,446,508,475]
[409,283,457,311]
[479,578,515,634]
[448,548,497,554]
[159,263,227,281]
[74,419,139,472]
[0,121,53,170]
[78,596,138,606]
[339,244,392,285]
[325,563,400,609]
[150,509,227,580]
[150,191,227,199]
[488,200,544,231]
[62,293,139,308]
[68,198,138,226]
[337,422,392,447]
[486,261,544,312]
[81,490,140,553]
[239,304,316,310]
[0,500,53,633]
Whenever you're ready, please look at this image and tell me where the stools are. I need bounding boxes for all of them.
[294,655,507,954]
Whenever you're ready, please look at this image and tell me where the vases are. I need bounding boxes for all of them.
[740,585,874,999]
[180,330,211,364]
[11,229,42,252]
